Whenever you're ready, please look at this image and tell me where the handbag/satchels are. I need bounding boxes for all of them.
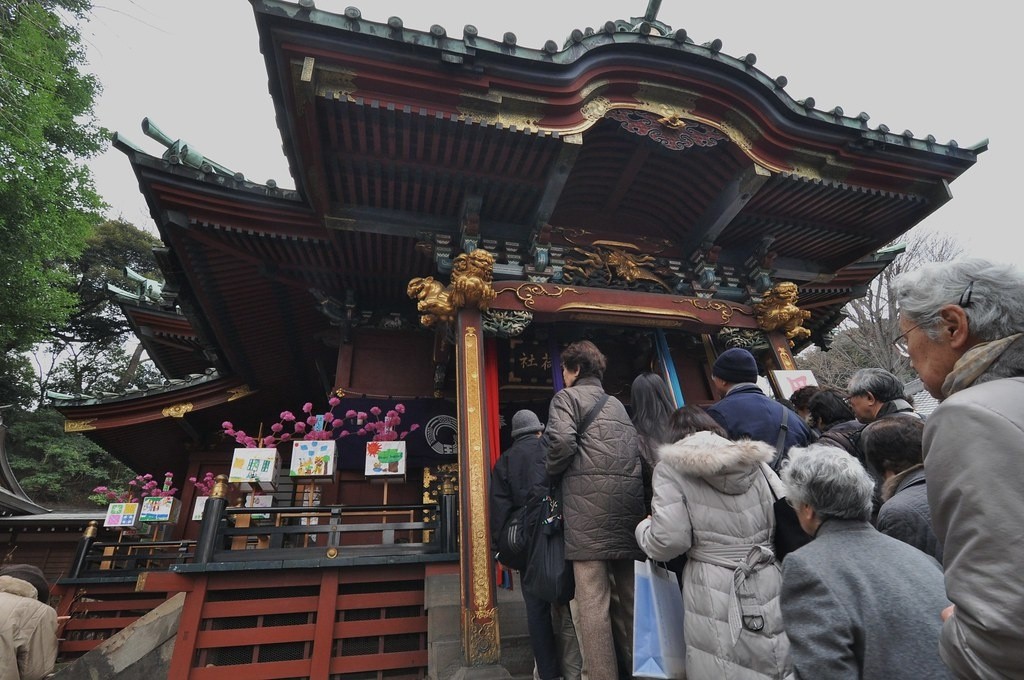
[522,495,575,601]
[632,554,686,679]
[499,506,530,569]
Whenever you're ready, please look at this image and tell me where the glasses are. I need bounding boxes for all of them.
[892,320,929,358]
[843,391,861,405]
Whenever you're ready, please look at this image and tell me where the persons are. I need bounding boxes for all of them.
[634,405,796,680]
[781,443,960,680]
[705,348,816,479]
[534,339,647,680]
[774,368,928,528]
[0,564,58,680]
[891,258,1024,680]
[490,409,576,680]
[633,372,688,591]
[861,417,944,565]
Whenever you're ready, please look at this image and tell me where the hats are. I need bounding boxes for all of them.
[0,564,49,603]
[510,409,545,437]
[711,348,757,383]
